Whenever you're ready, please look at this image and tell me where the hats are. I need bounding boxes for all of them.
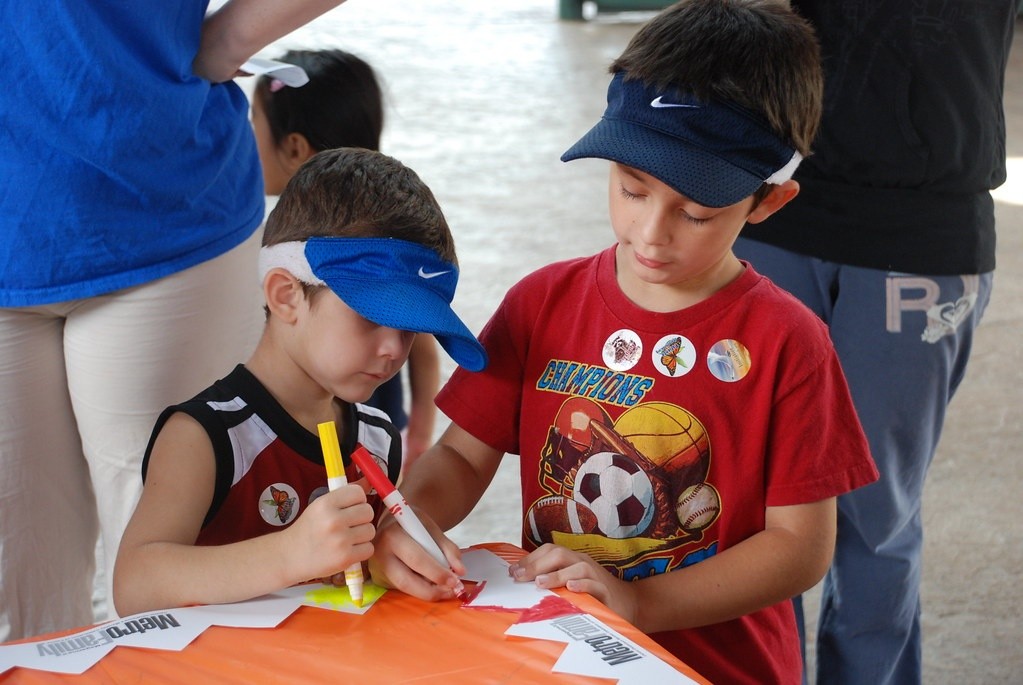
[560,73,805,209]
[257,236,488,373]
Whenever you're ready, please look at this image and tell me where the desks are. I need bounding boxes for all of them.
[0,543,714,685]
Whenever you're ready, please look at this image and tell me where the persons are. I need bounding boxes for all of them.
[0,0,348,640]
[732,0,1023,685]
[370,0,881,685]
[114,146,489,618]
[250,48,440,482]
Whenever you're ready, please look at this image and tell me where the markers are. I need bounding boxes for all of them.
[317,419,365,609]
[350,449,470,603]
